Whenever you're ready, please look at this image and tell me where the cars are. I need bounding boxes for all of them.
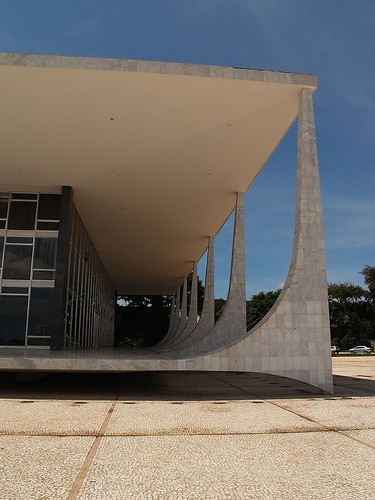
[331,346,337,352]
[349,346,371,355]
